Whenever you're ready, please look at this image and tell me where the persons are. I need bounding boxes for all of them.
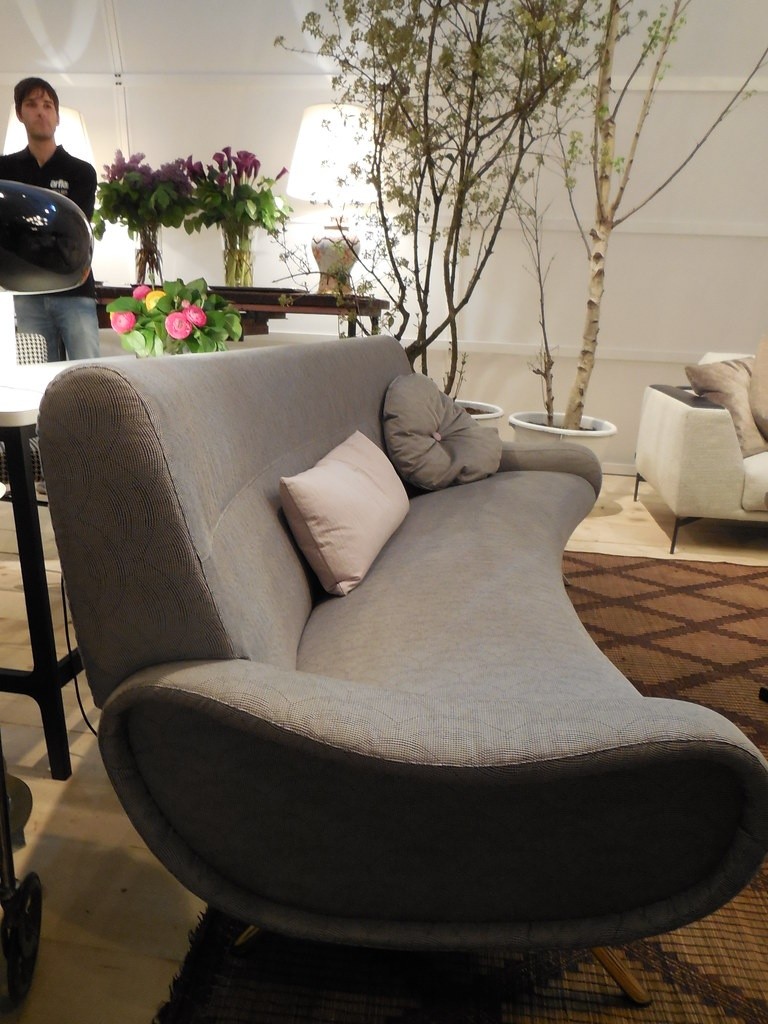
[0,77,102,365]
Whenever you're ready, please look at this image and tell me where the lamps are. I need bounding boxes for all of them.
[286,104,386,296]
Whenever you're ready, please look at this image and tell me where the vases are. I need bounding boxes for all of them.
[136,225,165,286]
[223,226,251,288]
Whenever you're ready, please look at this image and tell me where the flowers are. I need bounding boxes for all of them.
[105,277,245,357]
[92,150,194,240]
[183,147,288,240]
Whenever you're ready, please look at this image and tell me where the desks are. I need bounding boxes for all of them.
[94,286,391,338]
[0,355,83,780]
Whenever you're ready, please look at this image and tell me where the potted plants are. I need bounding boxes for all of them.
[271,2,601,428]
[507,0,768,465]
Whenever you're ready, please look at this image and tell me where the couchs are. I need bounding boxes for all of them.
[633,351,768,558]
[41,334,767,954]
